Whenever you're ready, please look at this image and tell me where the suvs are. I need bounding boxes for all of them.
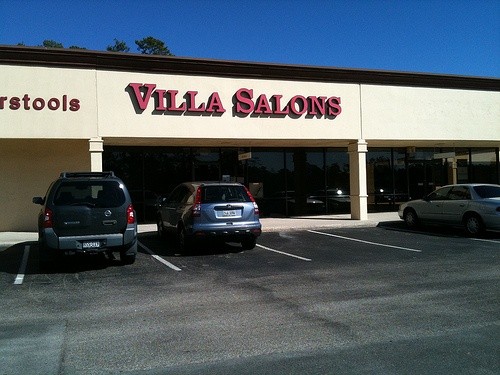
[32,171,137,264]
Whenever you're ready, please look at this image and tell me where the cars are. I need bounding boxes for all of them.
[399,183,500,236]
[155,182,262,250]
[272,190,350,215]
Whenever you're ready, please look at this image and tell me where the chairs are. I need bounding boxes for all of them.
[97,190,105,199]
